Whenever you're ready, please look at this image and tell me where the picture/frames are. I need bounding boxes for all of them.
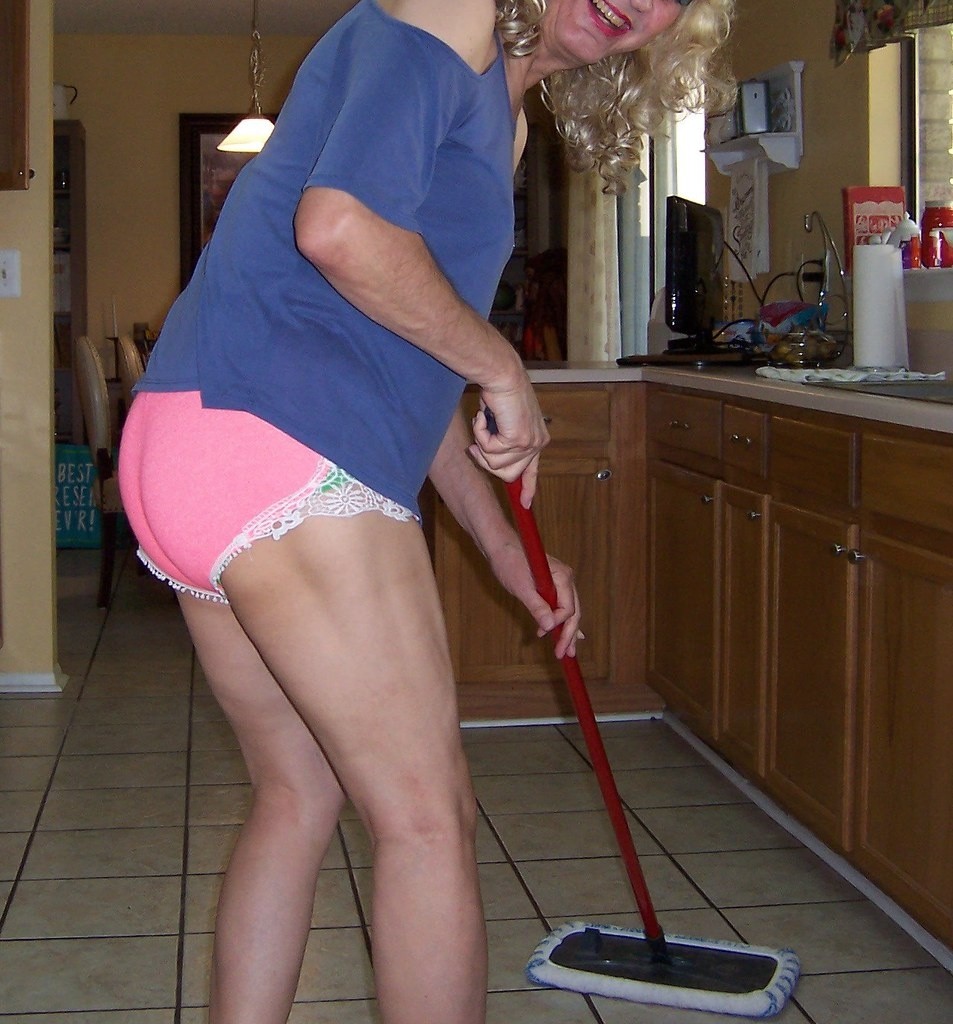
[177,112,279,292]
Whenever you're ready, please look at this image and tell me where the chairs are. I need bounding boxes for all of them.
[74,336,138,609]
[115,336,144,411]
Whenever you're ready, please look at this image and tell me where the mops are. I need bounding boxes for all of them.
[482,406,801,1018]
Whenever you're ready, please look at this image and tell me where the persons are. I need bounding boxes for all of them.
[115,0,745,1024]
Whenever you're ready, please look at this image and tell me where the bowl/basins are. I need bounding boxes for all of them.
[750,330,849,365]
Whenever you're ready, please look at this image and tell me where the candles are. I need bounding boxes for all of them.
[111,293,118,336]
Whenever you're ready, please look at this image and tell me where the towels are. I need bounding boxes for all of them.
[754,366,948,386]
[728,157,770,283]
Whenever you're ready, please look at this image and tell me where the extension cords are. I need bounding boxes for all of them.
[822,249,831,292]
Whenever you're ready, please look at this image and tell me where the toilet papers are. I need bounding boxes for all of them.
[853,245,909,370]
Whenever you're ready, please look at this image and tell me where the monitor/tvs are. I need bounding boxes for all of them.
[660,195,732,357]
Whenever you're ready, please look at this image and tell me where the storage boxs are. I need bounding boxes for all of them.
[840,185,906,275]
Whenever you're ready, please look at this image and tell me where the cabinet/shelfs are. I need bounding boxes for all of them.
[767,403,953,971]
[425,383,624,729]
[53,118,88,446]
[642,380,770,802]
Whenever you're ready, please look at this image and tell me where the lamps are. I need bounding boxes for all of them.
[215,0,275,152]
